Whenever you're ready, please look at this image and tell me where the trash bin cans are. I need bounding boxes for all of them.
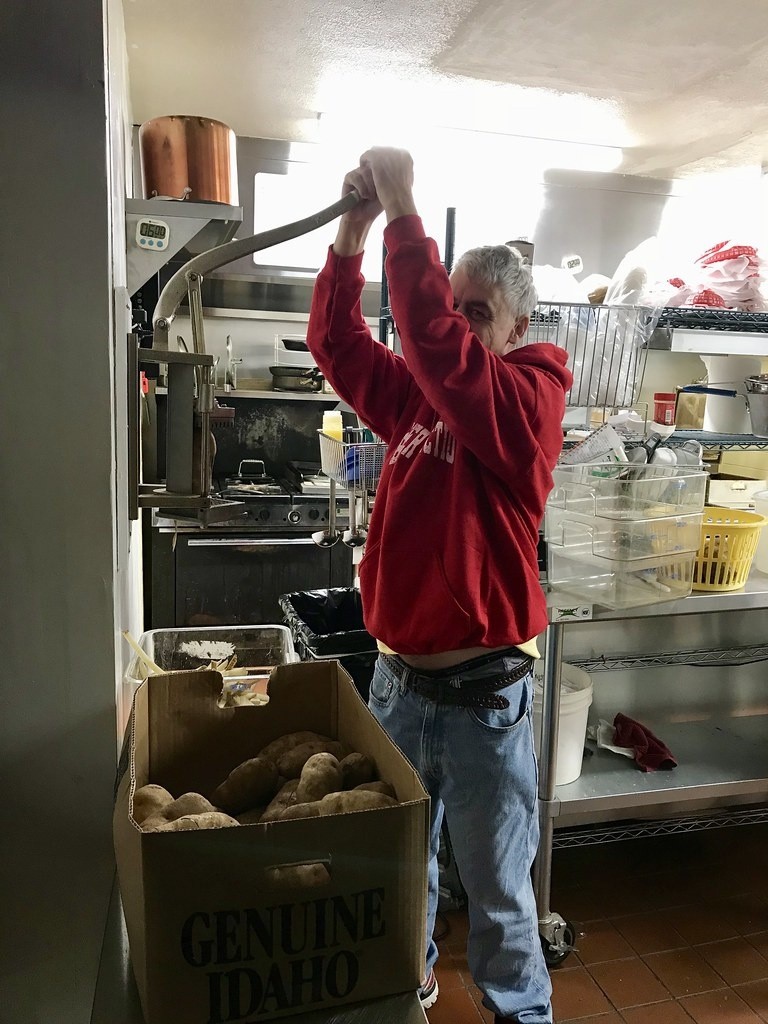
[277,585,381,705]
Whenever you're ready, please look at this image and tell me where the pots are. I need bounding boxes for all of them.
[684,386,768,439]
[269,365,325,392]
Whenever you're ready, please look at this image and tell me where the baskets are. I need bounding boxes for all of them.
[645,506,767,592]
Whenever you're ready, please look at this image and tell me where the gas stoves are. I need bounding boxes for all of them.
[152,460,350,527]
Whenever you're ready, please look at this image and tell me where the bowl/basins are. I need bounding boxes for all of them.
[744,373,768,393]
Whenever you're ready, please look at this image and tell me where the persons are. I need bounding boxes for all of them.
[307,146,572,1024]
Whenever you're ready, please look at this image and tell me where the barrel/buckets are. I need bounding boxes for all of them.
[529,659,593,785]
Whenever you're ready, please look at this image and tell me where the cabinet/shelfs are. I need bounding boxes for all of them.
[635,306,768,451]
[535,570,768,961]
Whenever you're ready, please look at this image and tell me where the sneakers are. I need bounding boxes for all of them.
[418,967,439,1009]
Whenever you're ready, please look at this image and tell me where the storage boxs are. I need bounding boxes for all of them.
[124,625,300,742]
[544,464,710,610]
[112,658,431,1024]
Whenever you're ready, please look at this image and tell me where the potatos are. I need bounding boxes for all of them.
[132,727,402,886]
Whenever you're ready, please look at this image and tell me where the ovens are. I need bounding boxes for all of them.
[152,529,352,629]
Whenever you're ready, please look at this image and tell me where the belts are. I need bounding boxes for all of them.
[379,651,532,710]
